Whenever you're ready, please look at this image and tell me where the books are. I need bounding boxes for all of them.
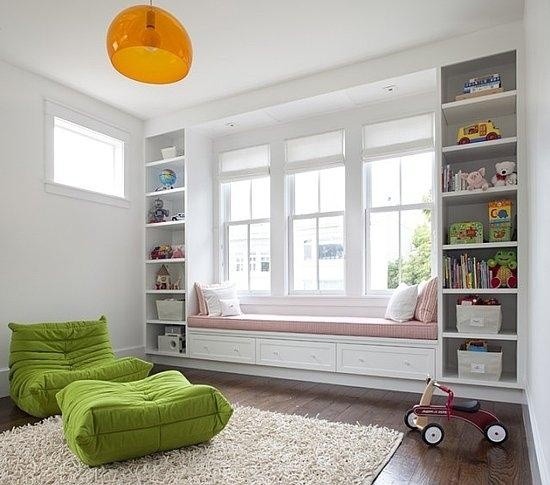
[441,253,492,289]
[440,165,466,192]
[453,73,505,102]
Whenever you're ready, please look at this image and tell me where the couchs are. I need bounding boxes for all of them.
[57,369,234,467]
[9,317,152,417]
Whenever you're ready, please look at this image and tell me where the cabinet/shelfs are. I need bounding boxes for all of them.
[141,127,185,355]
[440,50,521,386]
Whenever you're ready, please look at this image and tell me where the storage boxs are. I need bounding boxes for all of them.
[457,296,504,336]
[155,299,185,320]
[459,345,502,382]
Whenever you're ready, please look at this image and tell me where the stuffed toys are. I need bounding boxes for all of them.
[459,167,491,190]
[491,159,519,188]
[487,250,518,288]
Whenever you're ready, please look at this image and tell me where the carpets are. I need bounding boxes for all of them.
[0,404,403,485]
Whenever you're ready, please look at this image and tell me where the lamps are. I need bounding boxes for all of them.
[105,0,193,85]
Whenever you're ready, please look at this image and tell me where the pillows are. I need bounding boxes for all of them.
[197,281,218,315]
[384,284,416,320]
[205,283,243,316]
[416,279,438,323]
[189,333,438,397]
